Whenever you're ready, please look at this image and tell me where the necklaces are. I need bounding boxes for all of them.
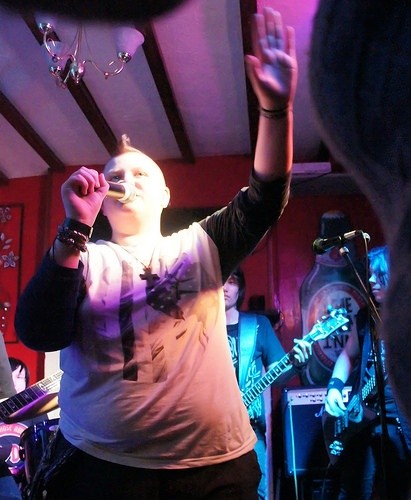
[111,239,160,289]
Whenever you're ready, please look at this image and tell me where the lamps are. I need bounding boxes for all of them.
[35,14,145,90]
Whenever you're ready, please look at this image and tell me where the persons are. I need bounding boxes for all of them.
[0,332,49,500]
[223,264,313,500]
[15,6,297,499]
[325,246,410,500]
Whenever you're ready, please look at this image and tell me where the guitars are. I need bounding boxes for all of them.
[241,304,352,408]
[1,368,63,422]
[321,370,390,465]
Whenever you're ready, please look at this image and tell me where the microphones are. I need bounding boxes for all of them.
[106,181,136,203]
[311,229,362,255]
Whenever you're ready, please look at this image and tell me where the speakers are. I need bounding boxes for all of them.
[283,384,352,477]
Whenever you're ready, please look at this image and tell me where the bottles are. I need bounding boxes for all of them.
[299,211,381,390]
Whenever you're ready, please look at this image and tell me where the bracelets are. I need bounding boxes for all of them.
[259,104,290,120]
[55,213,94,252]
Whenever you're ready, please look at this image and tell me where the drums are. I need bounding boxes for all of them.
[17,417,60,487]
